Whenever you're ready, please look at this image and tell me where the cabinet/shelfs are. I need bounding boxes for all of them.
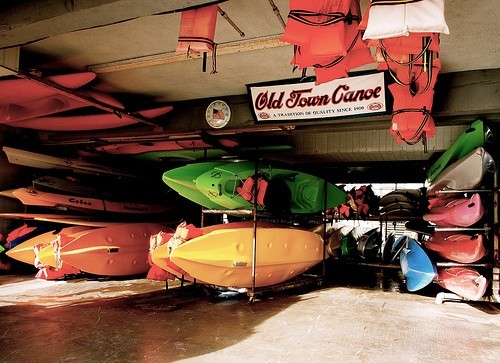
[428,167,500,306]
[193,144,329,306]
[314,182,428,293]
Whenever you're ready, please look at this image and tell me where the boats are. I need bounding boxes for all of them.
[0,65,495,303]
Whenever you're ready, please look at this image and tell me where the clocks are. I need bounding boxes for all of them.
[205,100,232,129]
